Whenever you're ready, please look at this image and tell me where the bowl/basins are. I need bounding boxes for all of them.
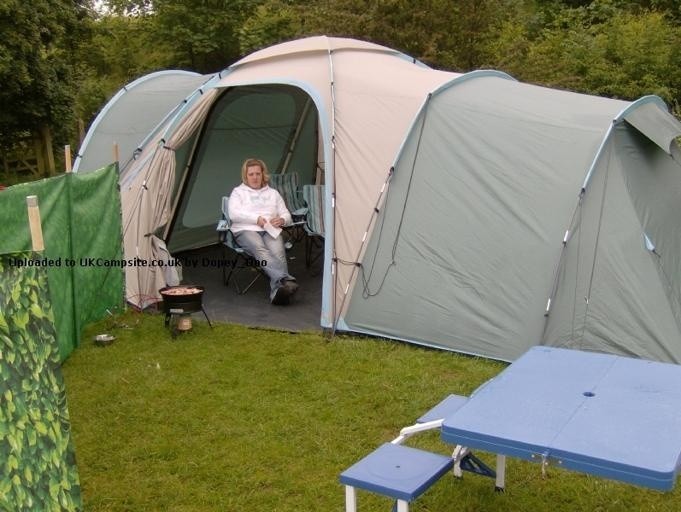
[95,339,114,347]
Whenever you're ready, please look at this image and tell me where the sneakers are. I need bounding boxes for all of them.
[270,281,300,305]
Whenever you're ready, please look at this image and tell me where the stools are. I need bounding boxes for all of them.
[416,394,508,492]
[337,433,457,510]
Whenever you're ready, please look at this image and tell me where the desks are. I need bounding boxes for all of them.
[442,339,680,492]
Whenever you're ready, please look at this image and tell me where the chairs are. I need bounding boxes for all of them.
[217,194,276,295]
[300,184,328,272]
[267,173,310,280]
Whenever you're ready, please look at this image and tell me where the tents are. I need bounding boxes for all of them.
[63,30,681,369]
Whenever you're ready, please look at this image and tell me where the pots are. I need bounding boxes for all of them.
[158,284,205,302]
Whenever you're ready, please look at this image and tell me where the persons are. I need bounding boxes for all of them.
[226,157,299,306]
